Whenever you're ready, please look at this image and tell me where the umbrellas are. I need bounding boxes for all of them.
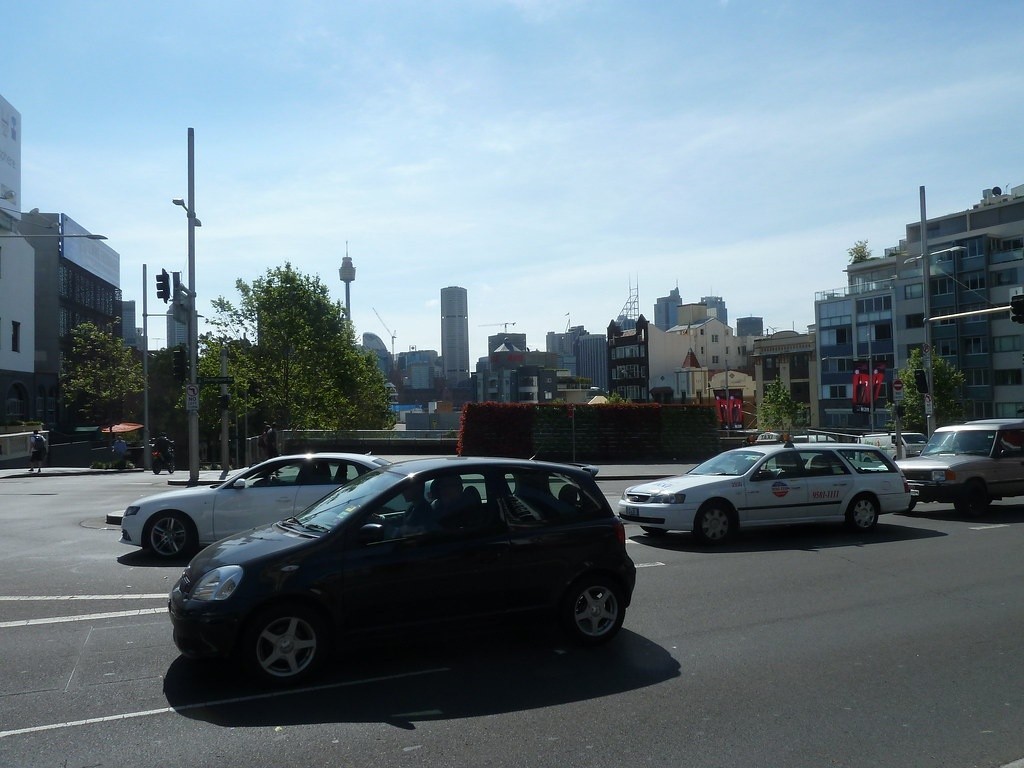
[101,423,144,432]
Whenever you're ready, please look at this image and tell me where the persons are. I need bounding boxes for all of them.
[154,432,173,474]
[114,436,126,457]
[29,430,47,473]
[398,474,477,535]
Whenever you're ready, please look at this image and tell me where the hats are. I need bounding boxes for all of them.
[159,432,166,436]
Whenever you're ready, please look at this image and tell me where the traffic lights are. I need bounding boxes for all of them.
[1010,293,1024,324]
[915,368,929,393]
[156,268,170,304]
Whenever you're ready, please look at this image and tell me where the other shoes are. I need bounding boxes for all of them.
[38,469,41,472]
[29,469,33,471]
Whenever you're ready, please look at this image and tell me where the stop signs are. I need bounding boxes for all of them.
[894,380,903,390]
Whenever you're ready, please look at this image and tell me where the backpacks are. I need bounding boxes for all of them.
[33,436,44,450]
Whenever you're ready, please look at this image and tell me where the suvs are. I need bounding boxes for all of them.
[879,418,1024,521]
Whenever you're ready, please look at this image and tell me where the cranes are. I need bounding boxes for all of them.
[479,321,517,333]
[371,305,397,363]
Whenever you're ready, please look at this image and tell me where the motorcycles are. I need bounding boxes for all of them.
[148,436,176,475]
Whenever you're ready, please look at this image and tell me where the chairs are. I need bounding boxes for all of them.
[293,464,349,485]
[425,475,575,533]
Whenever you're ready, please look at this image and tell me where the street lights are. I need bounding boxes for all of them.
[172,126,203,481]
[903,184,968,440]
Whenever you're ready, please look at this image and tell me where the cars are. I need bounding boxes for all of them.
[118,452,433,565]
[167,456,636,690]
[617,432,911,551]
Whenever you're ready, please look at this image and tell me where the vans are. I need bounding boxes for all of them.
[854,431,929,464]
[749,434,847,467]
